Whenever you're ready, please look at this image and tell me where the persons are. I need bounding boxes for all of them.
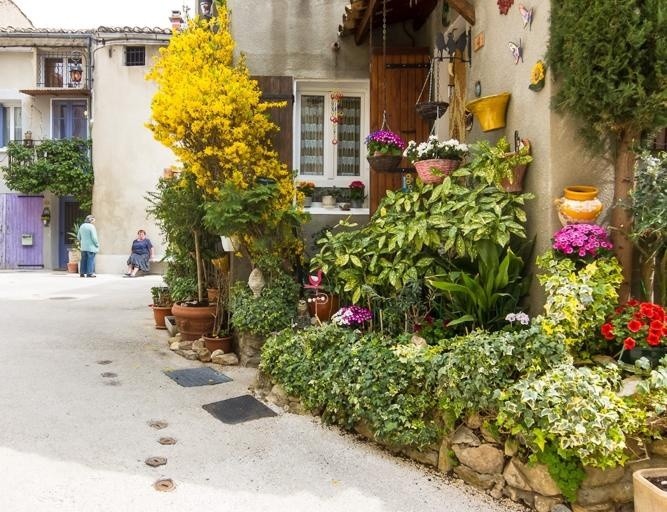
[77,214,100,278]
[124,229,155,277]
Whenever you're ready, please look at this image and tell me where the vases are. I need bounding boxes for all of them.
[65,262,78,274]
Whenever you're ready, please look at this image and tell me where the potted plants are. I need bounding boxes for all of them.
[143,159,233,356]
[66,223,82,273]
[282,87,530,323]
[630,467,667,512]
[550,183,667,369]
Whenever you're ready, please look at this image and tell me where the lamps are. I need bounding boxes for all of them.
[22,129,33,149]
[70,47,87,83]
[40,206,51,229]
